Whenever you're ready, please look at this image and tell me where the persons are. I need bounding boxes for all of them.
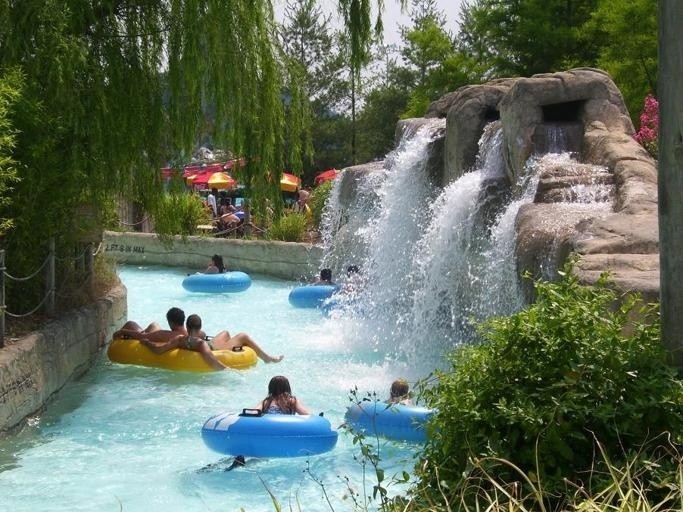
[196,375,311,475]
[347,265,359,278]
[296,184,312,213]
[113,308,206,341]
[139,314,284,370]
[205,187,240,240]
[312,269,337,286]
[195,253,226,274]
[383,378,416,406]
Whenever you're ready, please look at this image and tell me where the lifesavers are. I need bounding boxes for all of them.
[181,270,253,292]
[201,407,338,457]
[289,284,340,310]
[346,399,444,445]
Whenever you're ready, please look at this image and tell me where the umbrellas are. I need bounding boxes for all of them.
[281,173,302,192]
[315,167,340,186]
[187,170,234,188]
